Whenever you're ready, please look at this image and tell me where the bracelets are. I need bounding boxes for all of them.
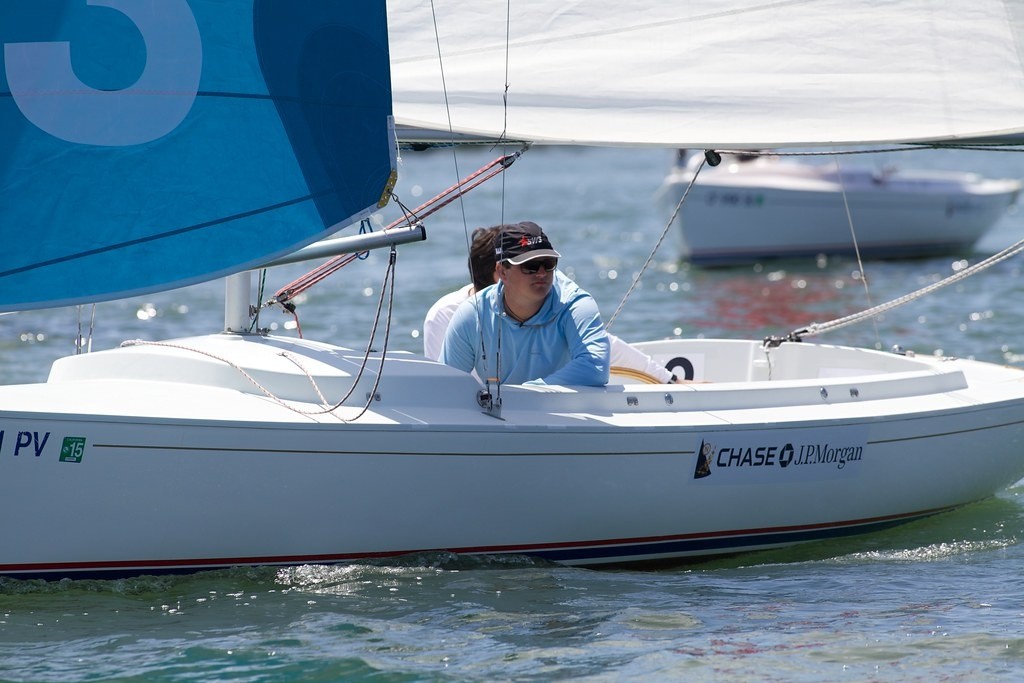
[668,375,677,384]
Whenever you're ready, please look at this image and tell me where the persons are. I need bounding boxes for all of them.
[439,221,611,386]
[424,225,714,384]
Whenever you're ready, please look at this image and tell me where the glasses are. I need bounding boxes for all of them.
[520,257,558,275]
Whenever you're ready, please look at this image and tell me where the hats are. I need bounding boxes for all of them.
[494,221,561,266]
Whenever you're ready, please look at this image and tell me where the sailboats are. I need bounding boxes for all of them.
[0,0,1023,576]
